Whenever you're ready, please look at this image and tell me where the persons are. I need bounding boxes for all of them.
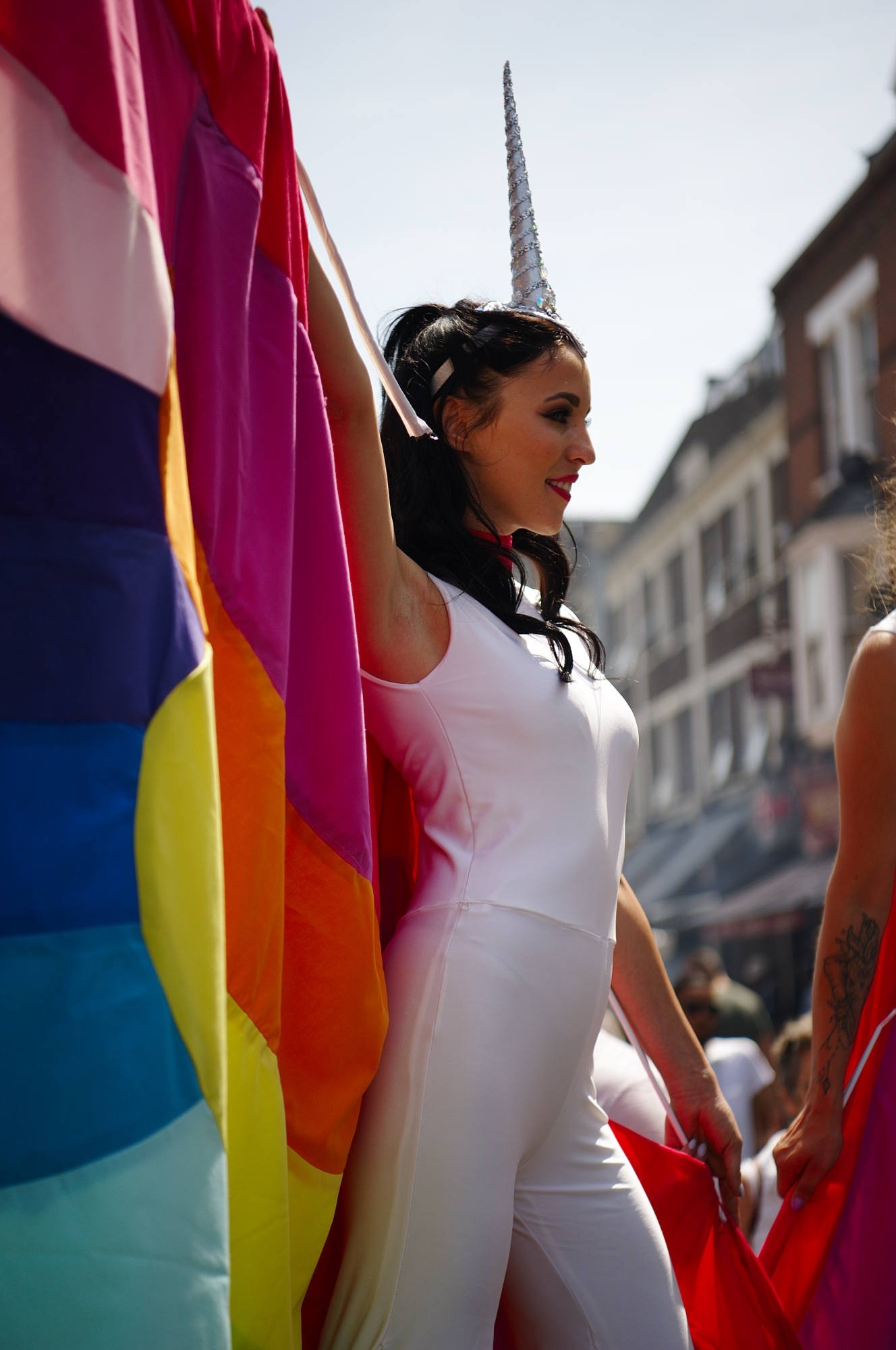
[306,242,743,1350]
[755,605,896,1350]
[588,949,812,1256]
